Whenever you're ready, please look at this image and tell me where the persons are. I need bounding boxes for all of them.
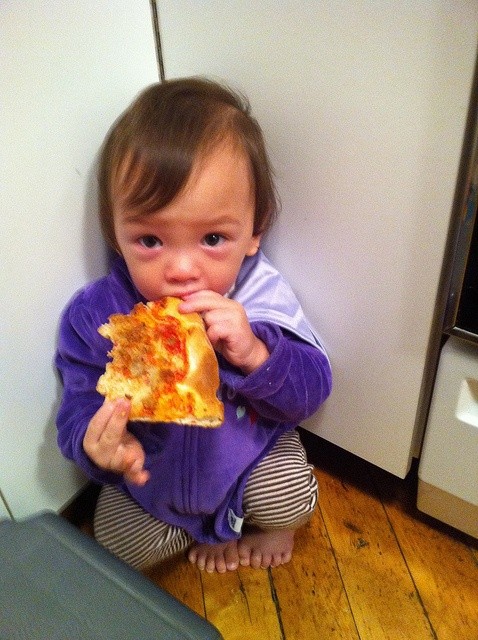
[50,74,333,573]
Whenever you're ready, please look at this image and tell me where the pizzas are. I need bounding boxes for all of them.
[94,292,227,432]
